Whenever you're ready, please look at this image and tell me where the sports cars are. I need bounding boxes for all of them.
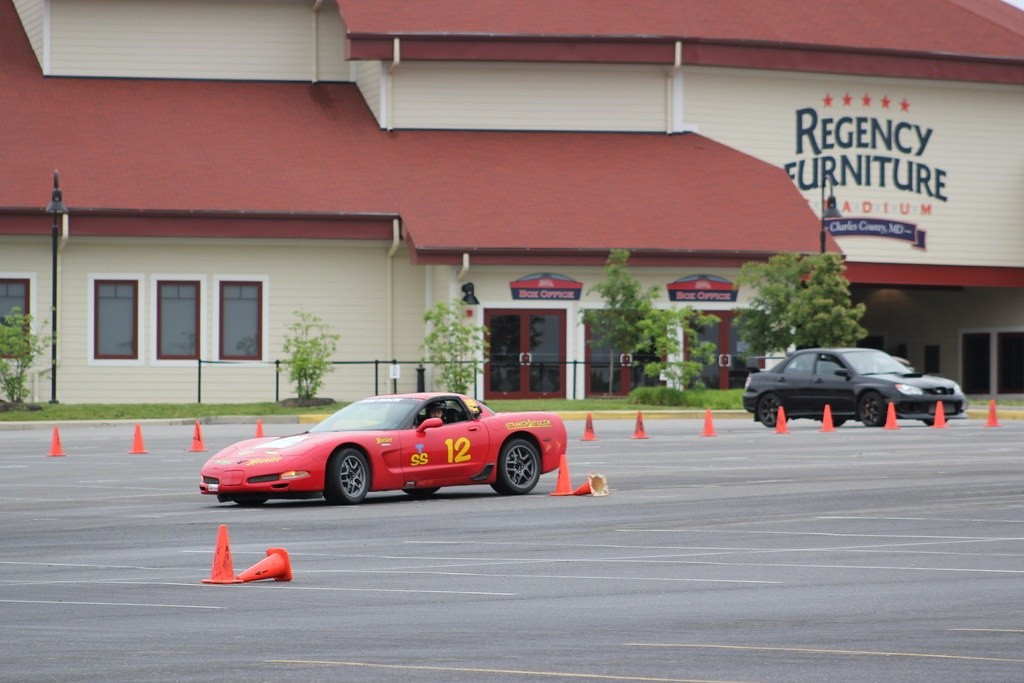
[197,390,568,504]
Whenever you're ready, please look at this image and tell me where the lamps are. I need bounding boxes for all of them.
[461,283,480,305]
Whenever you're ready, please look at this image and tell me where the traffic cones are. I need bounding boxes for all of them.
[929,398,950,428]
[881,402,900,429]
[128,424,147,453]
[256,419,265,438]
[549,454,572,496]
[984,400,1000,427]
[701,408,718,436]
[815,404,837,431]
[771,405,791,433]
[47,426,66,456]
[188,420,207,451]
[234,548,291,581]
[572,474,610,497]
[580,412,596,440]
[630,410,648,438]
[202,523,243,585]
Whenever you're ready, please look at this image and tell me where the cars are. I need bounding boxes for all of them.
[740,346,967,429]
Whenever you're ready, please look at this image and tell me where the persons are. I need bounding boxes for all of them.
[414,401,448,426]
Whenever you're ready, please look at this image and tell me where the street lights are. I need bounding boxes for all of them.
[42,169,69,401]
[819,175,844,249]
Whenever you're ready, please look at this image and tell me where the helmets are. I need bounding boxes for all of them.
[426,401,447,418]
